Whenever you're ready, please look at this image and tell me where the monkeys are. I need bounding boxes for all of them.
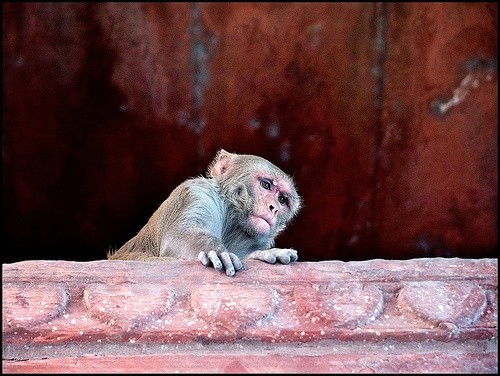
[106,148,303,277]
